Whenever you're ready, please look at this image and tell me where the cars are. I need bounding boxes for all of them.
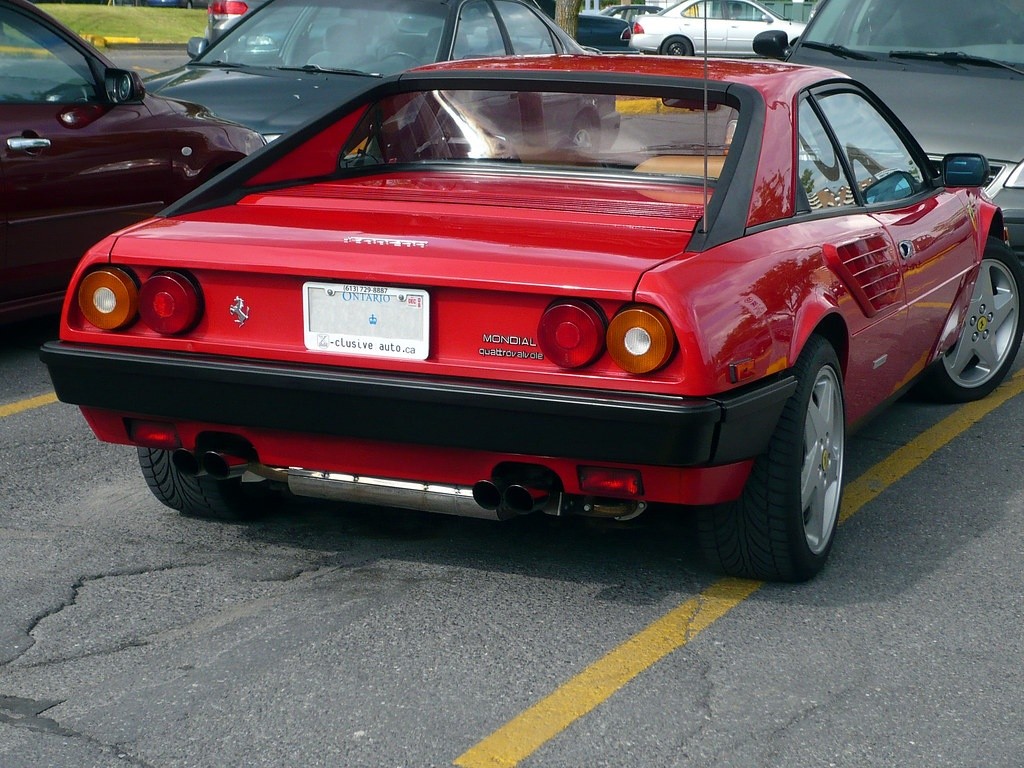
[518,0,640,55]
[139,0,622,187]
[40,49,1024,583]
[751,0,1024,270]
[594,4,665,35]
[629,0,810,59]
[0,1,269,334]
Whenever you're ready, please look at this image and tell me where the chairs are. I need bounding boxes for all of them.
[421,25,471,64]
[305,24,371,69]
[632,155,727,177]
